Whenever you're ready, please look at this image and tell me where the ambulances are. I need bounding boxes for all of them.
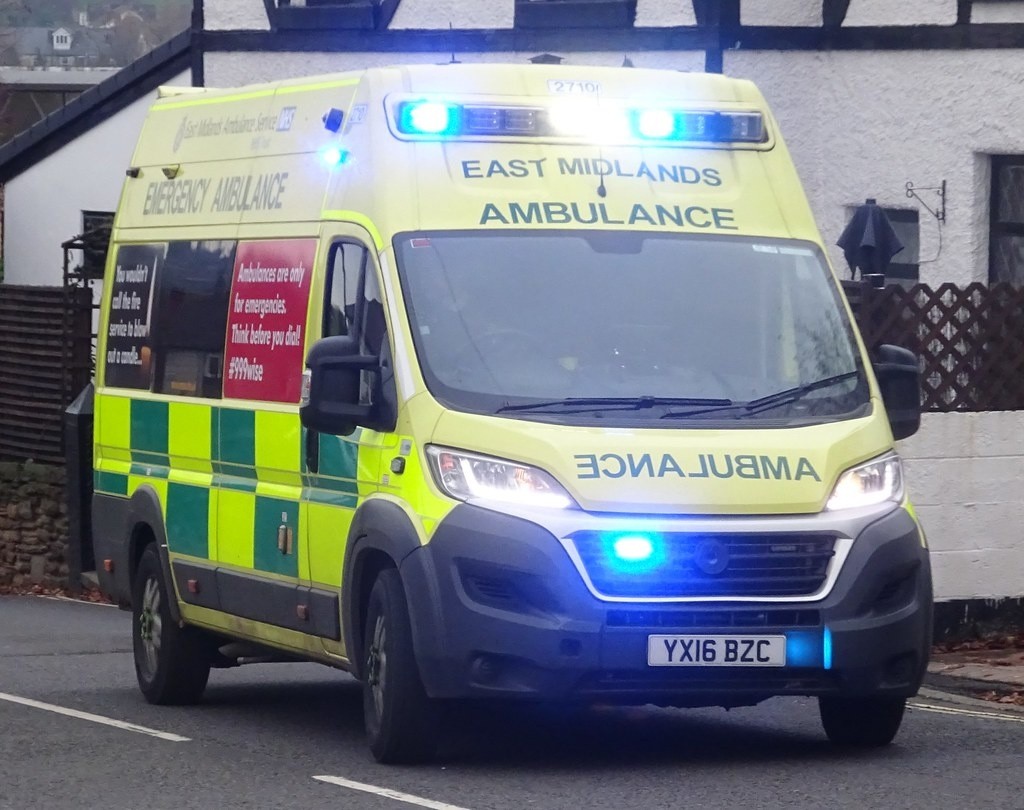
[91,62,933,764]
